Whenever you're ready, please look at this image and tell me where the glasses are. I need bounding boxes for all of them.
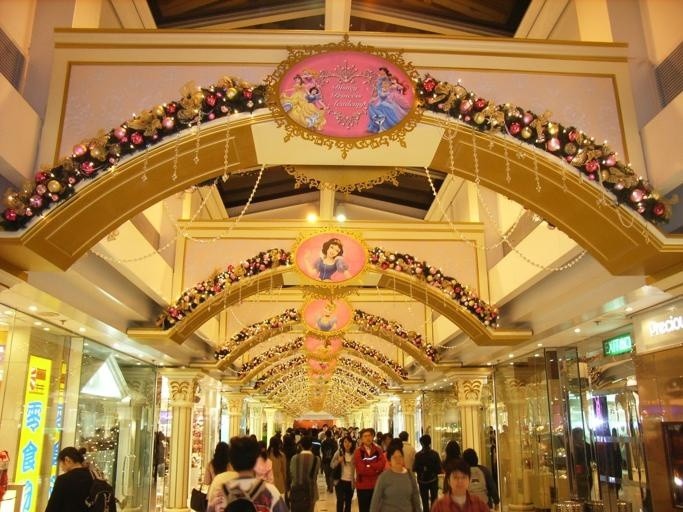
[449,476,468,480]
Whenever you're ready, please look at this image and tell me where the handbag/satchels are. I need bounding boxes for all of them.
[331,463,341,480]
[190,488,207,512]
[289,485,311,512]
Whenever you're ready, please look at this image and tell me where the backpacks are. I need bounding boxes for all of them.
[321,441,333,464]
[83,471,115,512]
[417,452,437,486]
[222,479,264,512]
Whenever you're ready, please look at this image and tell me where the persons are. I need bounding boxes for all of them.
[204,427,323,511]
[153,431,170,477]
[318,424,501,511]
[44,446,116,512]
[572,427,627,501]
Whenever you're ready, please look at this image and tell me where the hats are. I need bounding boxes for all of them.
[311,442,321,448]
